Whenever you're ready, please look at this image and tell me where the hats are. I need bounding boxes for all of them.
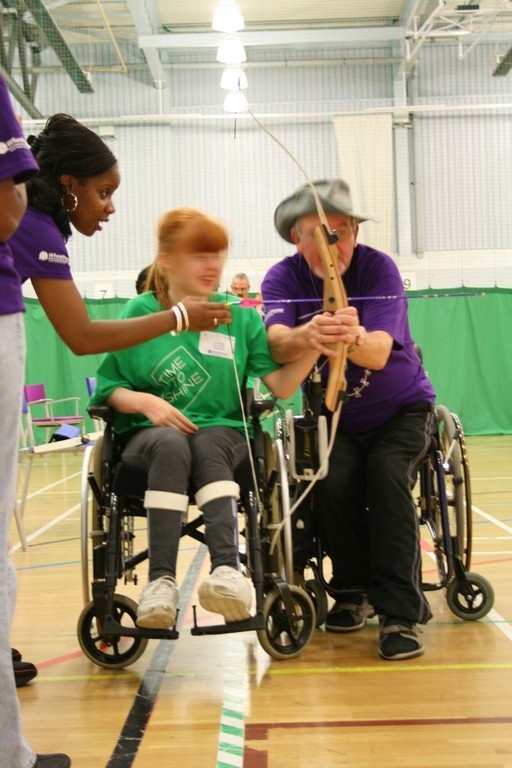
[272,176,383,245]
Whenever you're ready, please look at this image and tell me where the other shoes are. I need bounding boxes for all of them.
[10,649,38,686]
[33,753,70,768]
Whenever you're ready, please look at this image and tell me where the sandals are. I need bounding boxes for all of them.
[378,614,423,660]
[324,594,375,632]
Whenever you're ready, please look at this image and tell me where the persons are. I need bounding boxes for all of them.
[135,263,169,295]
[0,72,72,768]
[232,274,261,300]
[0,113,232,688]
[262,180,437,660]
[86,210,365,629]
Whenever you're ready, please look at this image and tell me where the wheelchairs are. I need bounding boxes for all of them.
[277,346,497,630]
[74,388,333,670]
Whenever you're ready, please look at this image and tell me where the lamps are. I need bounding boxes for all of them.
[207,0,249,115]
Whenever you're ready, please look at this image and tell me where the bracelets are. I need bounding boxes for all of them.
[346,335,359,354]
[171,306,182,336]
[177,302,190,331]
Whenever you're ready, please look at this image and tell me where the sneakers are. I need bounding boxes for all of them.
[197,566,256,622]
[134,576,181,630]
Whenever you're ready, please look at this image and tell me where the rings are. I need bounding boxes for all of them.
[214,318,218,325]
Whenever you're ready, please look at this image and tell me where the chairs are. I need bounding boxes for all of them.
[13,377,100,550]
